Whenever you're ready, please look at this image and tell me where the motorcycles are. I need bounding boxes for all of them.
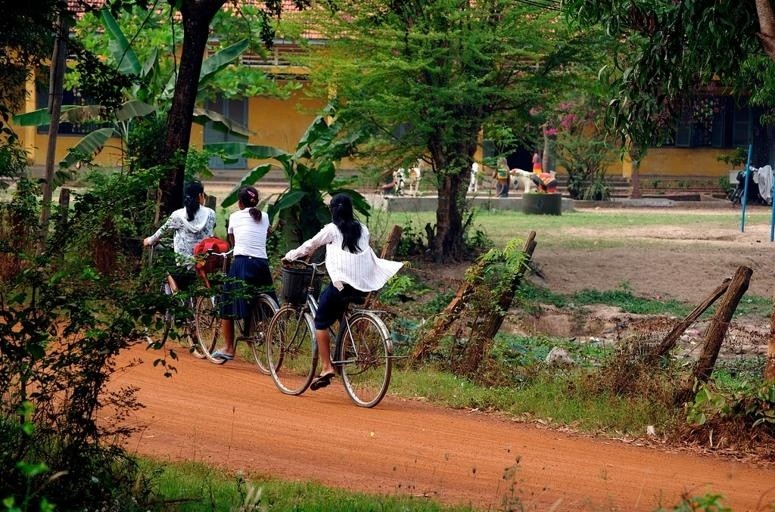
[727,165,775,206]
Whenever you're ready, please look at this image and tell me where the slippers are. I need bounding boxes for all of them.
[212,351,234,360]
[310,373,335,391]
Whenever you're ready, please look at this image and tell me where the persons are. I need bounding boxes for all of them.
[208,184,281,362]
[530,152,548,193]
[470,161,479,193]
[280,193,405,390]
[142,180,218,328]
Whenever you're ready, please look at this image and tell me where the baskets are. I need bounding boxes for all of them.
[194,236,230,276]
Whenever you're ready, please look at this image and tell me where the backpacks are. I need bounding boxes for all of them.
[279,267,325,304]
